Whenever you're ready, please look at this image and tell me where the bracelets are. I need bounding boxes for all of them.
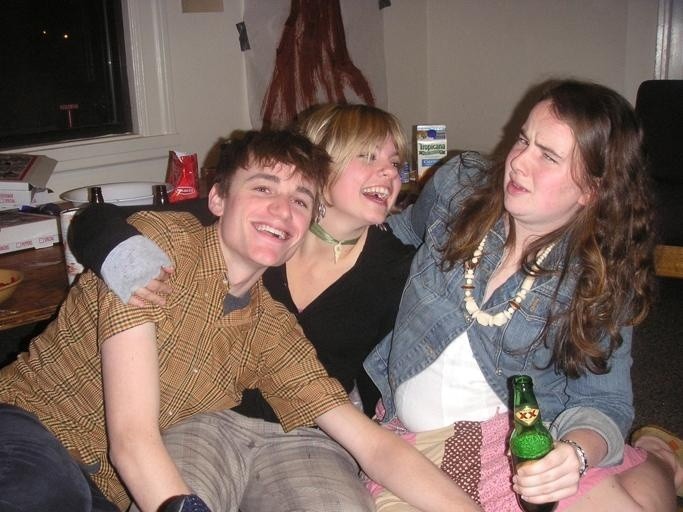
[559,437,588,476]
[157,494,211,511]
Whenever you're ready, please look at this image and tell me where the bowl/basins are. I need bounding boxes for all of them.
[58,179,173,208]
[0,268,23,304]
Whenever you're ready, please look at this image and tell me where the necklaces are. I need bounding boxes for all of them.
[308,221,361,266]
[461,232,560,327]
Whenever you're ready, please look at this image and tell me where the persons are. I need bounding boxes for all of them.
[361,79,681,512]
[68,105,414,511]
[0,129,482,511]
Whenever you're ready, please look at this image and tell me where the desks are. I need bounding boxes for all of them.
[0,244,69,332]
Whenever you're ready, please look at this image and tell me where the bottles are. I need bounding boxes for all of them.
[153,185,169,209]
[400,161,410,184]
[507,375,558,511]
[90,186,103,204]
